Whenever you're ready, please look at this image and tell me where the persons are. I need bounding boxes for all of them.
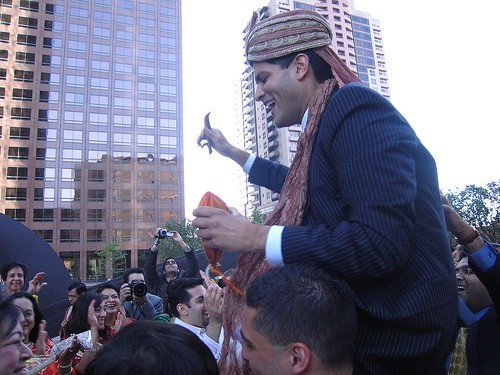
[438,192,500,375]
[451,228,491,264]
[166,278,224,365]
[239,264,360,375]
[0,262,47,301]
[59,282,86,341]
[192,11,454,375]
[7,291,81,375]
[64,291,123,374]
[98,283,135,345]
[0,295,34,375]
[119,268,164,321]
[144,228,199,316]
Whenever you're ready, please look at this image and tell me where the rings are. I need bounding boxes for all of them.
[69,349,74,352]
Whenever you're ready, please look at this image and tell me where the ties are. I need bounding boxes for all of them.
[447,326,471,375]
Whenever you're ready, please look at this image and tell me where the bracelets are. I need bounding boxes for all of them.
[153,241,159,245]
[57,361,72,369]
[58,367,72,375]
[454,226,480,245]
[84,349,97,353]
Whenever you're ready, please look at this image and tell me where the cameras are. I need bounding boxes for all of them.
[125,280,148,297]
[158,229,175,239]
